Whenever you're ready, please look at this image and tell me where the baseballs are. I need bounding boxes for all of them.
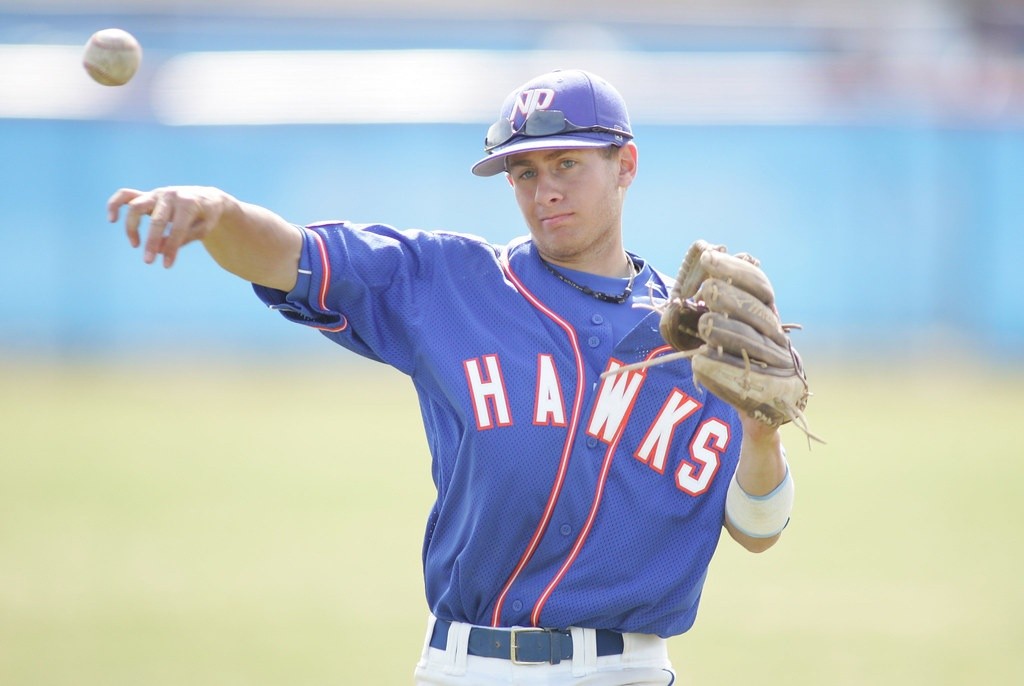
[83,26,143,87]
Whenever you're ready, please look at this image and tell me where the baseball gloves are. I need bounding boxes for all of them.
[658,240,809,427]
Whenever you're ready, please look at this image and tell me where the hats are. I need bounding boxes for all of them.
[472,70,633,177]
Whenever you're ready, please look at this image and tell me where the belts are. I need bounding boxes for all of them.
[428,620,624,665]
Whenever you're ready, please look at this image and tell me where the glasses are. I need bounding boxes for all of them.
[484,110,633,153]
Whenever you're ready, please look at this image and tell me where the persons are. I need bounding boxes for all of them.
[106,64,810,686]
